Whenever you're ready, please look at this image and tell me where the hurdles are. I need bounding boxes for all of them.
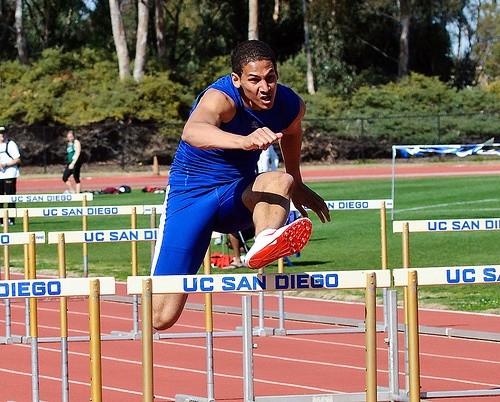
[0,193,500,402]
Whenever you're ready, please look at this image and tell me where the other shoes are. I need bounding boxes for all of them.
[7,218,16,226]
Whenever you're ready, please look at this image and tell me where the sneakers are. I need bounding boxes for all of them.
[245,218,312,269]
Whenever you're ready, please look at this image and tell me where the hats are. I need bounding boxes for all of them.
[0,125,9,134]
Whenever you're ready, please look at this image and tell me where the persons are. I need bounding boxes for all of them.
[60,130,92,193]
[0,125,24,227]
[153,40,332,331]
